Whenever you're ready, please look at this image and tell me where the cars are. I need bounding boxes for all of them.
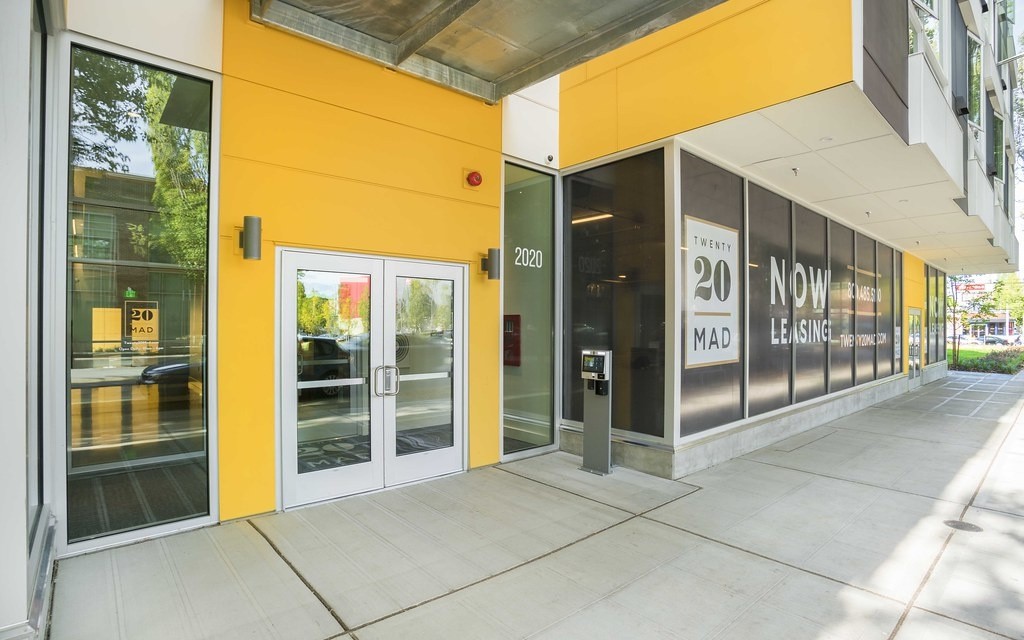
[946,335,966,343]
[976,336,1008,345]
[141,330,445,401]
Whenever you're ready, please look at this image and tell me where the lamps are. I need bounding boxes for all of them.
[481,248,500,279]
[240,215,261,260]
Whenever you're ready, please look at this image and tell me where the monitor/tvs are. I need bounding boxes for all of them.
[581,351,609,381]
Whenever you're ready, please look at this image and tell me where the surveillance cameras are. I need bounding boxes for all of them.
[545,154,553,164]
